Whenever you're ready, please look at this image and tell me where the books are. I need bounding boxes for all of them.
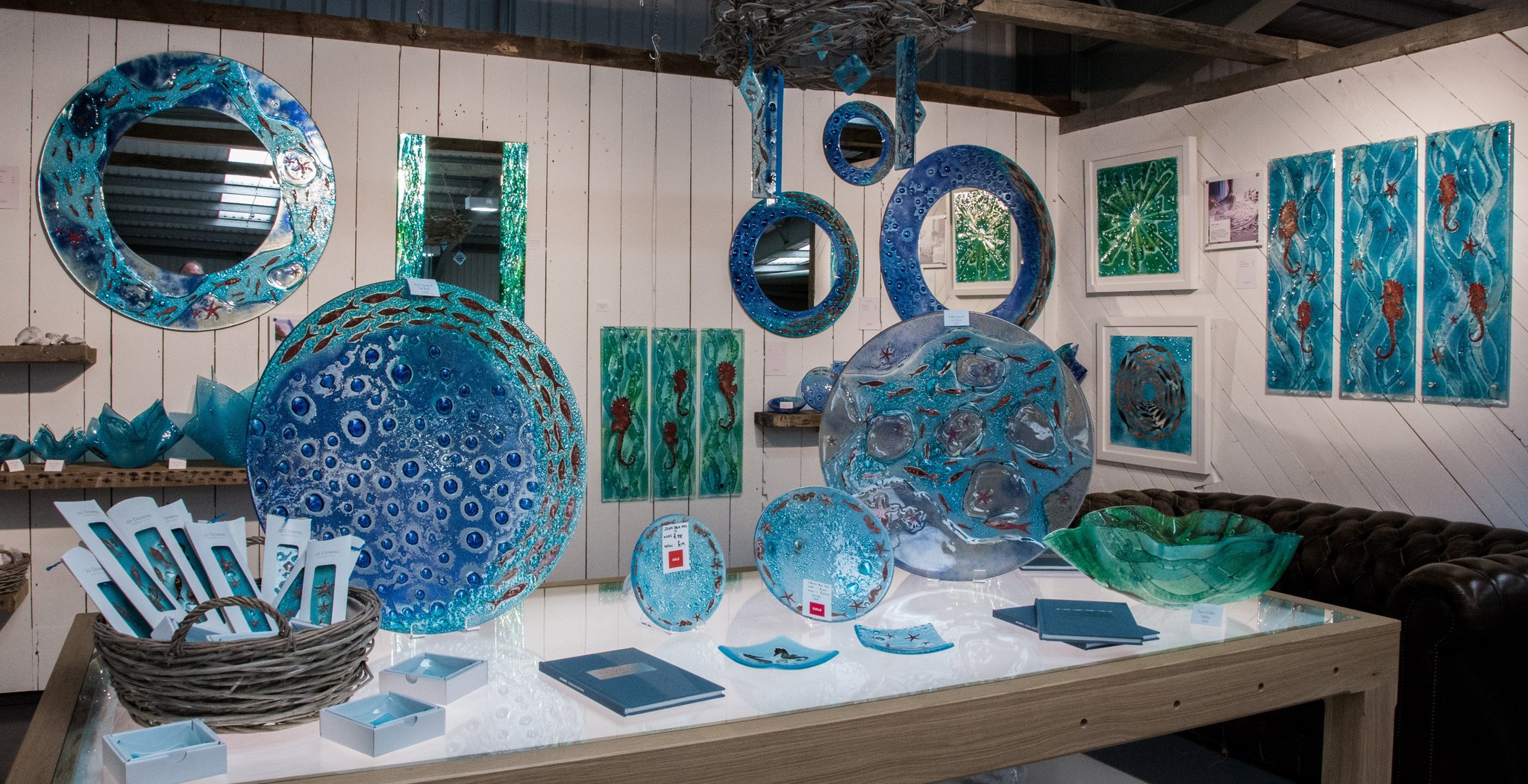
[539,647,726,718]
[992,598,1161,650]
[1018,558,1081,572]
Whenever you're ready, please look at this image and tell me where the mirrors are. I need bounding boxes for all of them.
[879,144,1056,330]
[36,51,336,330]
[822,101,896,186]
[396,133,530,320]
[728,191,860,337]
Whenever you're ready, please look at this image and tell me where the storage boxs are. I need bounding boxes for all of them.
[320,692,445,757]
[102,718,227,784]
[379,652,488,704]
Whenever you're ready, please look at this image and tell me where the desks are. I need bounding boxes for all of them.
[5,553,1402,784]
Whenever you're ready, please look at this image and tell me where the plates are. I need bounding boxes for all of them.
[182,375,259,466]
[0,434,33,464]
[630,514,726,631]
[31,424,89,465]
[819,310,1094,580]
[244,280,587,634]
[1043,505,1304,610]
[854,623,954,655]
[1054,342,1088,382]
[801,367,840,411]
[768,397,806,413]
[718,634,839,670]
[754,486,895,622]
[82,399,184,468]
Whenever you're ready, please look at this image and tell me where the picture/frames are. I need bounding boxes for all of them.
[1095,317,1212,474]
[951,189,1020,295]
[1081,135,1201,293]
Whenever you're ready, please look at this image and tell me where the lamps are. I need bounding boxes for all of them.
[464,193,499,212]
